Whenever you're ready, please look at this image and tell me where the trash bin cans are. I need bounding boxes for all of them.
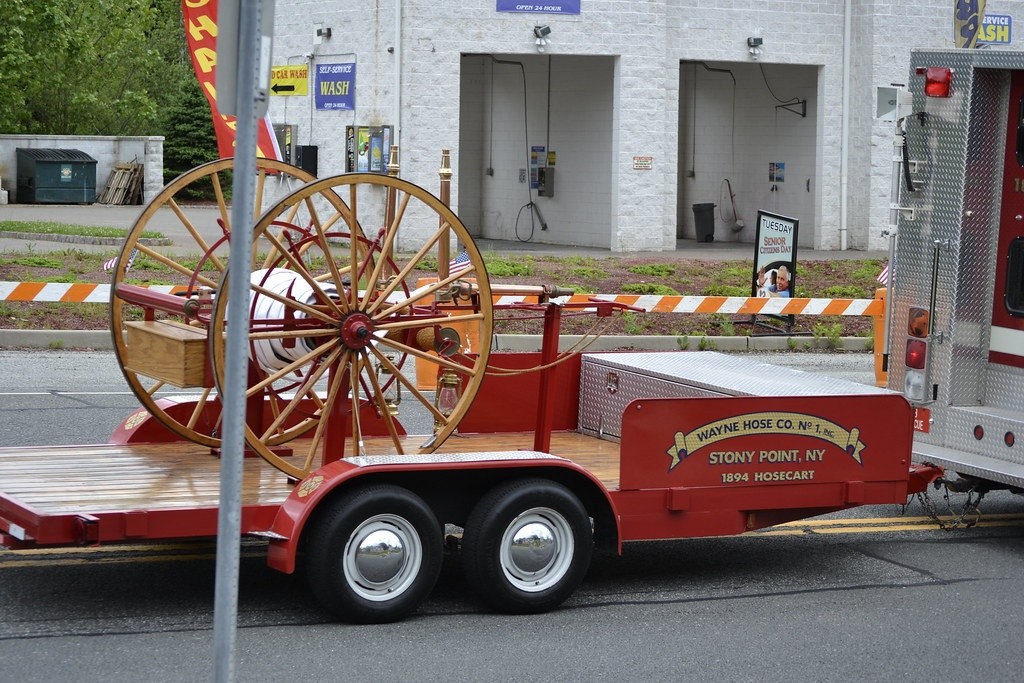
[15,147,98,205]
[692,203,717,242]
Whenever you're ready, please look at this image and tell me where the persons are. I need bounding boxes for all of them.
[756,265,790,298]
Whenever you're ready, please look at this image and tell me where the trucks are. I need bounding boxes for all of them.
[882,47,1024,495]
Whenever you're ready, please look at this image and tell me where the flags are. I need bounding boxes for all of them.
[878,257,896,287]
[103,248,137,272]
[449,250,471,276]
[181,0,282,176]
